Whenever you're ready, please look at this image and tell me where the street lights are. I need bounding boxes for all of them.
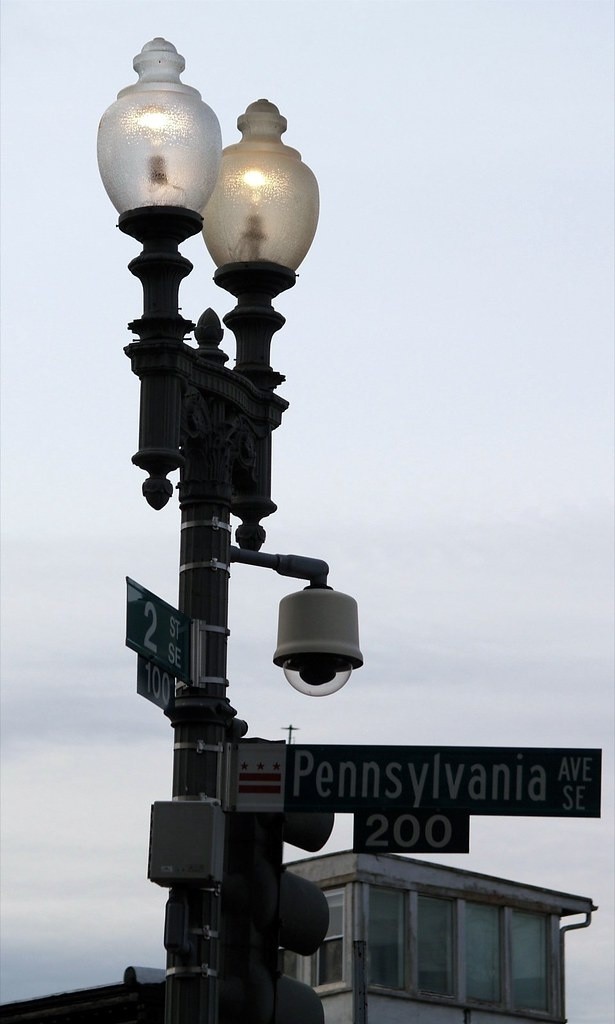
[93,37,365,1024]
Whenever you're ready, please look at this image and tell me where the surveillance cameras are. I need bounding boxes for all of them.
[273,589,364,696]
